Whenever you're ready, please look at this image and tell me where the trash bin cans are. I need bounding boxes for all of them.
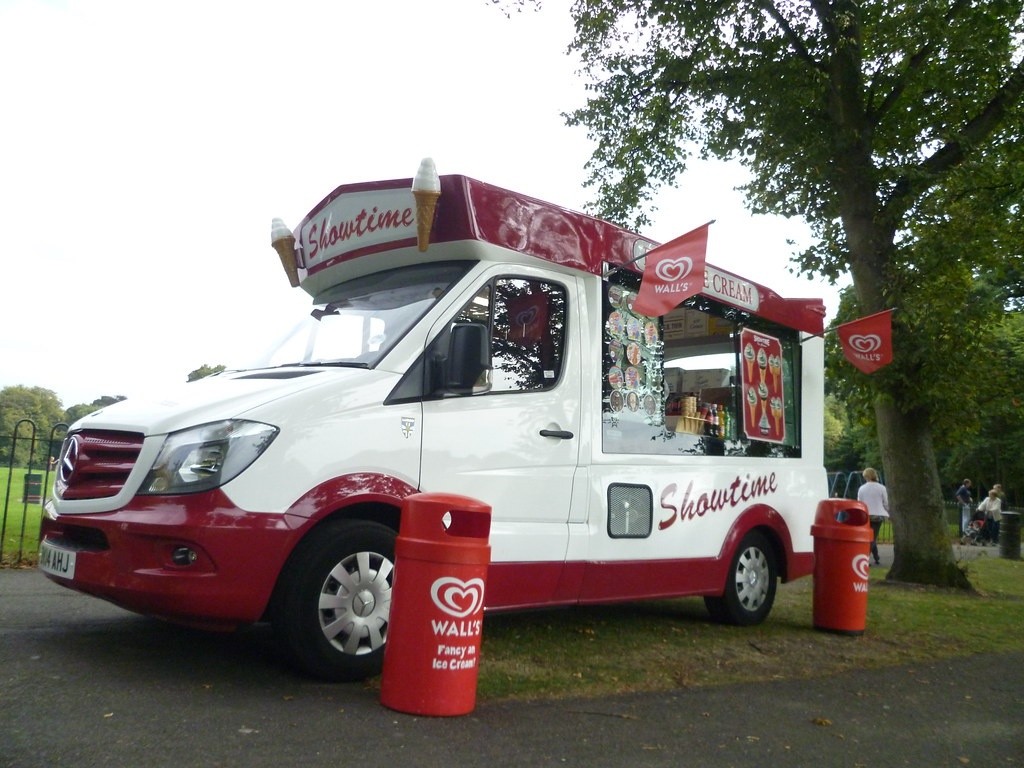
[998,512,1021,559]
[381,492,492,715]
[810,498,874,636]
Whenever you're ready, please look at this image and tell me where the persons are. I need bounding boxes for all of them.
[857,468,890,566]
[955,478,972,544]
[975,490,1001,546]
[994,484,1009,511]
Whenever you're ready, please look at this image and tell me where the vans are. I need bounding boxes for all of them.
[33,157,830,672]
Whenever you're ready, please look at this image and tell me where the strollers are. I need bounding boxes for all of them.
[960,509,990,546]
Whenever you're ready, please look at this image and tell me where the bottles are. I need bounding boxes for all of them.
[706,404,736,439]
[672,394,689,410]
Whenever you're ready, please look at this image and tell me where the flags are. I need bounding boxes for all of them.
[631,223,708,318]
[837,311,892,375]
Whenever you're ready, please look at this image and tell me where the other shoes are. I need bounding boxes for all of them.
[874,561,881,566]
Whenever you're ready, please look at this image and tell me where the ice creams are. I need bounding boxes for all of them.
[271,217,301,287]
[411,158,441,252]
[744,342,782,436]
